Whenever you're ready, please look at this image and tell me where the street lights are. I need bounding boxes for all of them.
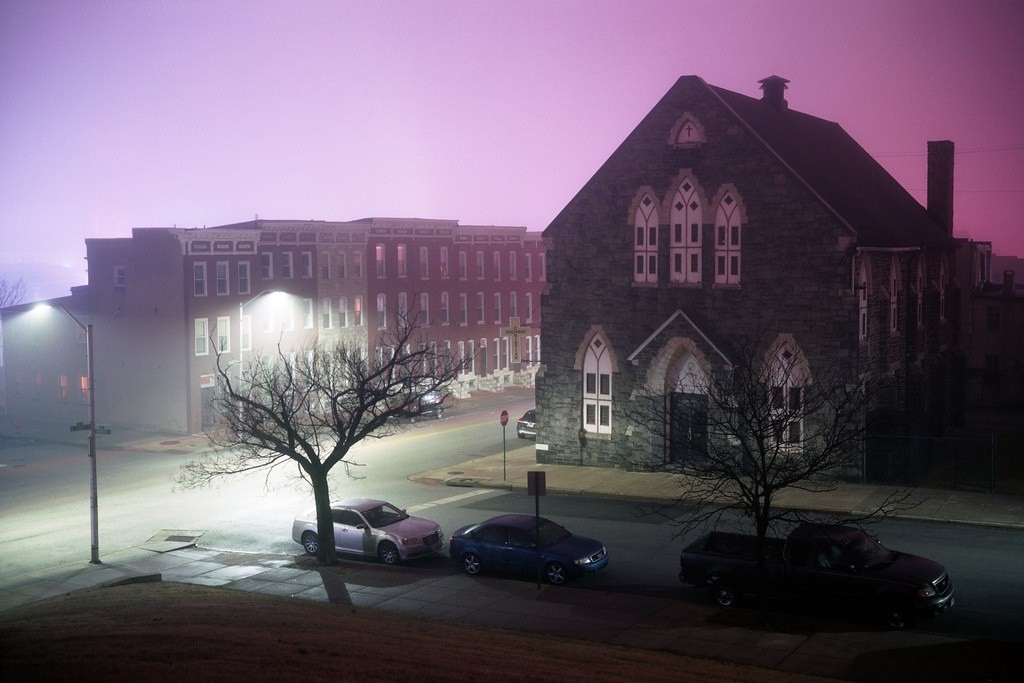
[31,303,102,564]
[236,289,286,441]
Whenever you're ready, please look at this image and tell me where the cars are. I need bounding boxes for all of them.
[516,409,537,439]
[449,514,611,586]
[387,392,445,423]
[291,497,446,566]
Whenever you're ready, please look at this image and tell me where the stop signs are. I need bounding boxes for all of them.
[500,411,509,426]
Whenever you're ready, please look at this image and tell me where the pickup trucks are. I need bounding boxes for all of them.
[679,521,954,630]
